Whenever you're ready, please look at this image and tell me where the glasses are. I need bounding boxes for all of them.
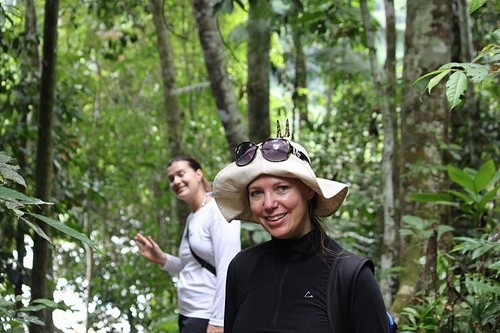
[232,138,311,167]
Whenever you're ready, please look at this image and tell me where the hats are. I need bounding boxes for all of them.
[212,138,349,223]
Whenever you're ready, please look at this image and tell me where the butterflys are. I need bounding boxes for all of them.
[276,118,290,138]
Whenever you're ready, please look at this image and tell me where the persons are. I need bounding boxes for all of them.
[211,136,393,333]
[135,154,242,333]
